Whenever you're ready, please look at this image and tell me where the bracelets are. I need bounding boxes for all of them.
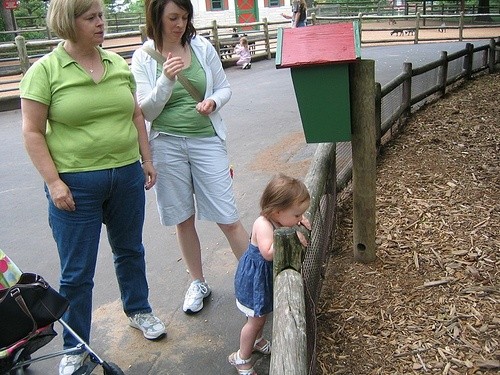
[141,159,153,165]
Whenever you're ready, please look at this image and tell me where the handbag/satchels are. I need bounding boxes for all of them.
[0,272,70,347]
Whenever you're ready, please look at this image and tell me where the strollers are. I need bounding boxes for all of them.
[0,249,126,375]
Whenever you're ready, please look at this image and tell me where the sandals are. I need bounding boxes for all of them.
[253,336,272,354]
[228,348,257,375]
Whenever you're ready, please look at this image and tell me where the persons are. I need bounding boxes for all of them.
[281,0,299,28]
[294,0,306,27]
[227,171,314,375]
[132,0,252,316]
[17,0,168,375]
[234,37,252,69]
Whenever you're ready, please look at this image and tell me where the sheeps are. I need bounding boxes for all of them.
[390,29,415,36]
[388,19,396,25]
[437,23,447,33]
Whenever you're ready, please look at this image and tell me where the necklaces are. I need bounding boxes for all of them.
[68,52,103,73]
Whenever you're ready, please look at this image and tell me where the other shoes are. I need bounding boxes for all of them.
[247,63,251,69]
[242,63,249,69]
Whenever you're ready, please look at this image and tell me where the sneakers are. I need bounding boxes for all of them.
[128,313,167,339]
[58,353,84,375]
[182,279,212,314]
